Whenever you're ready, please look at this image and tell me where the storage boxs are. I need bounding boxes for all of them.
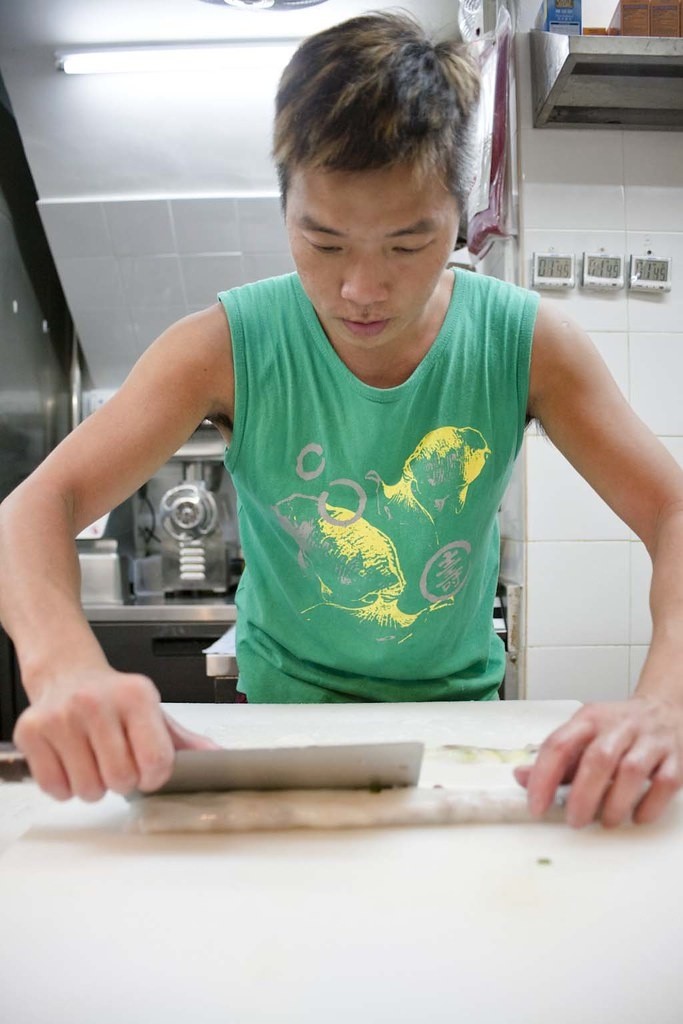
[534,0,683,37]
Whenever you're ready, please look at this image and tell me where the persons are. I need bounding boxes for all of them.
[0,10,683,828]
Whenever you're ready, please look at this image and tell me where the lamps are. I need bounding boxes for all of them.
[54,46,305,77]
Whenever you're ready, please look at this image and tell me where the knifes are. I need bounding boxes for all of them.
[0,735,426,791]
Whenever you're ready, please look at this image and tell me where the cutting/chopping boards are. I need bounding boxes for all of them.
[0,674,682,1024]
